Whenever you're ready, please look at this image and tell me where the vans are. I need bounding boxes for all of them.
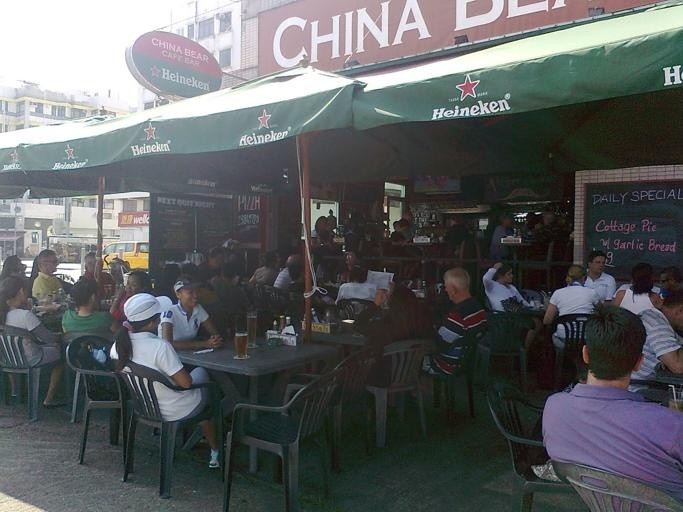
[101,241,148,272]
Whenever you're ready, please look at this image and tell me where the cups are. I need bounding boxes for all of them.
[533,297,541,310]
[233,331,249,357]
[245,310,257,348]
[668,386,682,410]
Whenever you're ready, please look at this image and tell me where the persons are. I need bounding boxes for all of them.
[32,250,69,332]
[83,252,113,300]
[110,271,152,320]
[482,250,678,394]
[1,276,65,405]
[351,281,418,367]
[109,293,221,469]
[311,211,569,289]
[2,255,32,298]
[157,277,225,371]
[409,268,489,400]
[62,278,131,401]
[542,301,682,511]
[197,239,305,301]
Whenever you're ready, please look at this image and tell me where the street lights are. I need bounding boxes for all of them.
[12,188,30,257]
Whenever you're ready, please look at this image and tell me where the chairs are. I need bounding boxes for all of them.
[0,243,683,509]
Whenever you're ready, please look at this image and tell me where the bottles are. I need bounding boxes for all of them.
[421,280,427,296]
[310,308,321,324]
[544,291,551,310]
[52,288,64,303]
[273,315,290,330]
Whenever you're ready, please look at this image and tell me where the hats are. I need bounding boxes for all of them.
[70,279,97,296]
[124,293,173,323]
[173,280,202,292]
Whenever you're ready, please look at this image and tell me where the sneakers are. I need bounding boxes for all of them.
[209,449,220,468]
